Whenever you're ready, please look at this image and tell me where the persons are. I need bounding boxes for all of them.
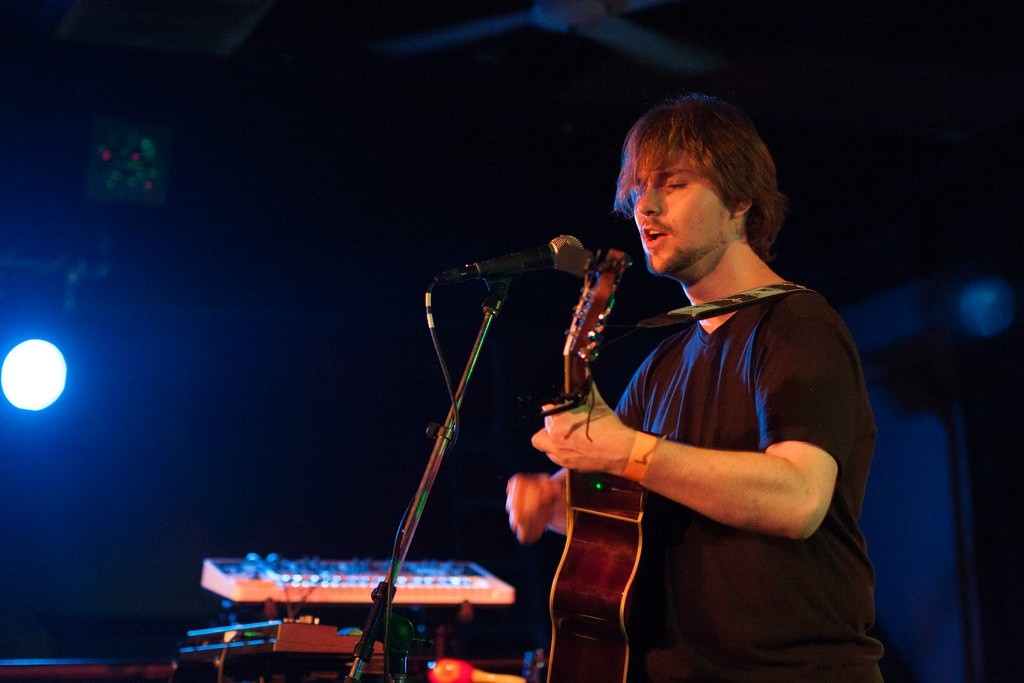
[505,97,884,683]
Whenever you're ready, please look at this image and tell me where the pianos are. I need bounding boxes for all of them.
[200,550,518,610]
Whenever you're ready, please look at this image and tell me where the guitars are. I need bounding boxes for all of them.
[539,247,645,683]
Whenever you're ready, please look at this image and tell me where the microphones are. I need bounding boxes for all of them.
[435,235,583,284]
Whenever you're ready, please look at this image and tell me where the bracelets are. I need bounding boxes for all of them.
[624,431,657,484]
